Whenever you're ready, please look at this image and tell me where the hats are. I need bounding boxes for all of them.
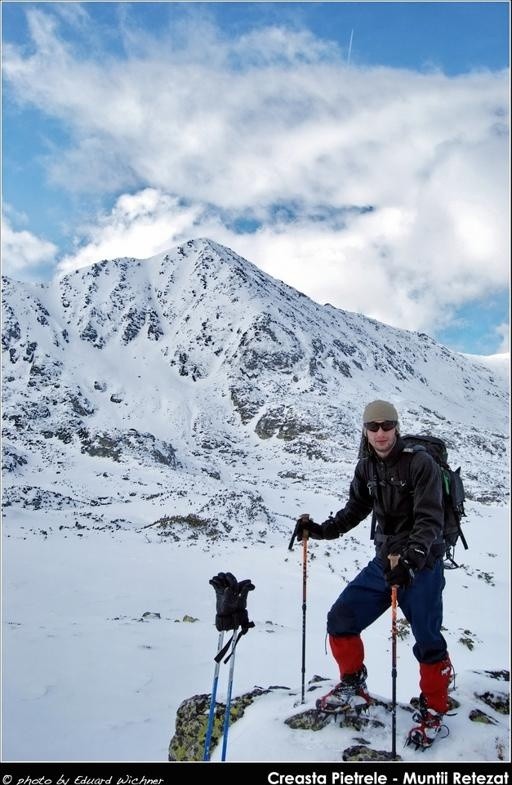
[363,400,398,423]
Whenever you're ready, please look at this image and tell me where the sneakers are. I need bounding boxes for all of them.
[409,709,444,748]
[316,681,370,714]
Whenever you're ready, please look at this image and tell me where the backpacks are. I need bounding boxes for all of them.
[367,435,467,551]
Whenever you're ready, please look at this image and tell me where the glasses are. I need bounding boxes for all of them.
[363,421,398,432]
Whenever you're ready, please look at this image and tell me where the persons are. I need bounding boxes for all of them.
[284,400,455,748]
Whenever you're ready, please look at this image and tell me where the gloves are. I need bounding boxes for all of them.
[209,572,255,632]
[295,516,339,541]
[383,558,418,591]
